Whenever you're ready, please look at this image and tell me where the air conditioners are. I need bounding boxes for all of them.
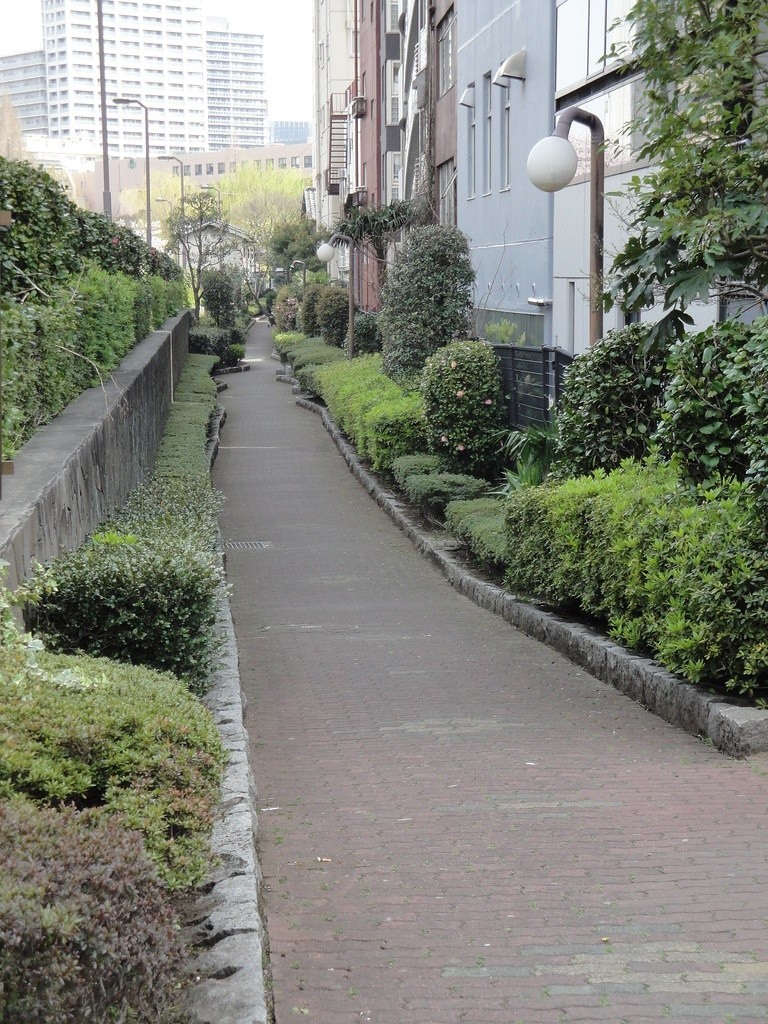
[352,100,367,117]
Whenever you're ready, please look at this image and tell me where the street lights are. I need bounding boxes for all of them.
[289,259,306,299]
[113,98,152,249]
[200,184,222,271]
[154,197,173,220]
[156,156,187,268]
[526,106,604,348]
[317,233,356,361]
